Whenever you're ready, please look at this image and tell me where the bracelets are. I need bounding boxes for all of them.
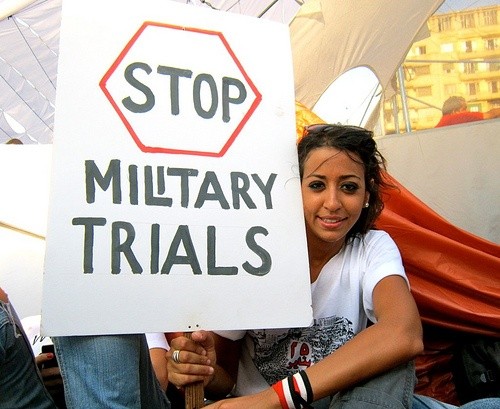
[272,369,313,409]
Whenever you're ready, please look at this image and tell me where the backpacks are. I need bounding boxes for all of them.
[455,334,500,404]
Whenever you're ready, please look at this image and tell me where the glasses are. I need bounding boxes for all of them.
[302,123,374,148]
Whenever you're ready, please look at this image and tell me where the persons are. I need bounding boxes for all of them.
[0,286,170,409]
[165,123,424,409]
[433,96,500,128]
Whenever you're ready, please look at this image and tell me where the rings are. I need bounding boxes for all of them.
[172,350,182,364]
